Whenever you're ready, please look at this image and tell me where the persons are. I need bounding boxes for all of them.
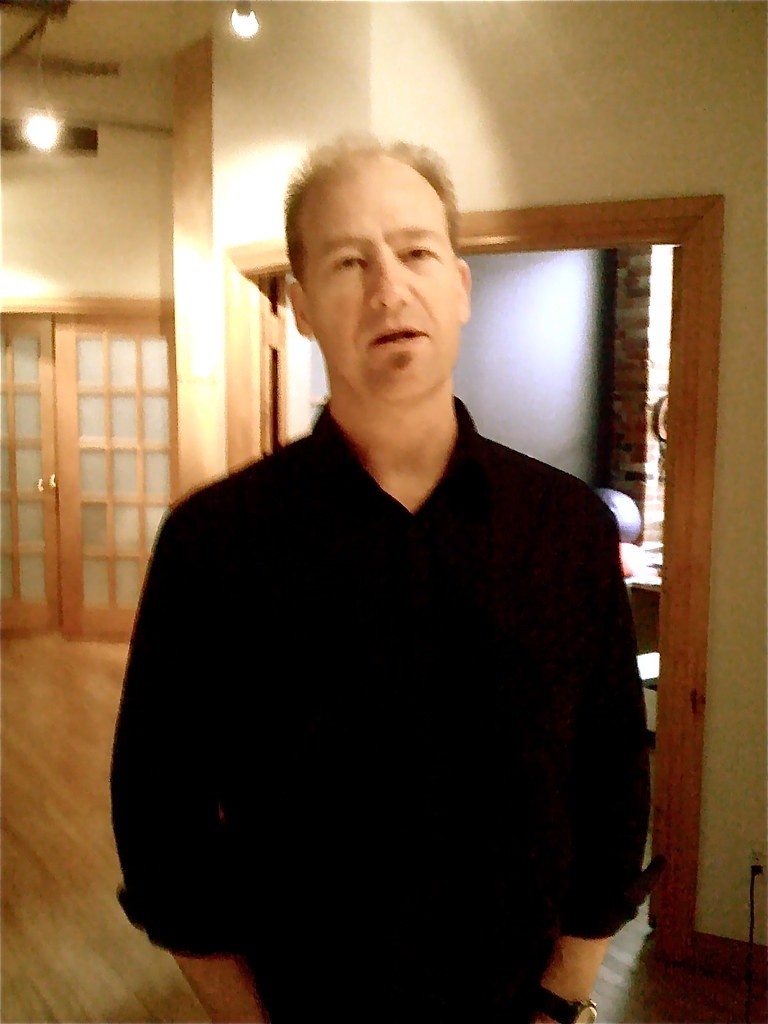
[112,137,651,1024]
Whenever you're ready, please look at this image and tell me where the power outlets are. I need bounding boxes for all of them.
[747,850,768,882]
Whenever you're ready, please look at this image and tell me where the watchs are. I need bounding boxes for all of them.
[534,984,599,1024]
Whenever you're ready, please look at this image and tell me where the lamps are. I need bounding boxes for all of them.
[24,18,63,151]
[224,1,268,38]
